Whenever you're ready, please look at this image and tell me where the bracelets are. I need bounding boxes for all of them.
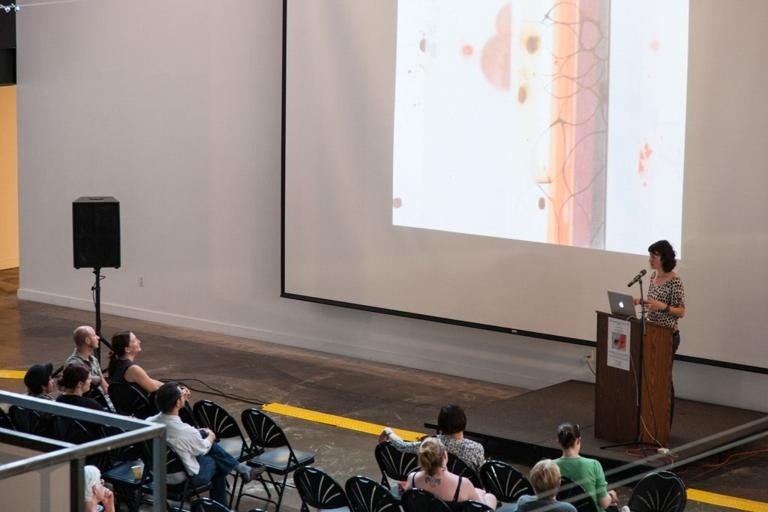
[662,304,670,314]
[384,430,392,440]
[607,493,613,504]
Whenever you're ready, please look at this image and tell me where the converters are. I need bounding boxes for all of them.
[658,447,669,455]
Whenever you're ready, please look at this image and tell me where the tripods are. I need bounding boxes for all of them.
[51,271,116,379]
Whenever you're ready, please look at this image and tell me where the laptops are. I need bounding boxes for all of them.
[608,291,636,317]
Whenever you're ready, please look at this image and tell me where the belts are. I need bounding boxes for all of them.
[673,330,679,336]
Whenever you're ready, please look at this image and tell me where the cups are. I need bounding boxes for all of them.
[131,466,142,480]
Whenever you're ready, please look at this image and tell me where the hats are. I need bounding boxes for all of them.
[23,362,53,392]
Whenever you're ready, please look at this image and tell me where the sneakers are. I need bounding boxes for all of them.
[241,465,266,484]
[621,506,630,512]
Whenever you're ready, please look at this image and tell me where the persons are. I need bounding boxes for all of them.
[83,464,117,512]
[619,341,626,350]
[21,362,57,402]
[611,339,620,350]
[56,363,105,410]
[108,330,191,415]
[63,326,116,412]
[552,422,619,511]
[144,382,265,511]
[403,436,498,512]
[633,240,685,431]
[379,404,484,475]
[512,458,577,511]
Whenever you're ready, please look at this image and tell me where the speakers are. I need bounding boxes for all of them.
[72,196,121,269]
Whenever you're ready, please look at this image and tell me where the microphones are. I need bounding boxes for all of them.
[628,269,647,287]
